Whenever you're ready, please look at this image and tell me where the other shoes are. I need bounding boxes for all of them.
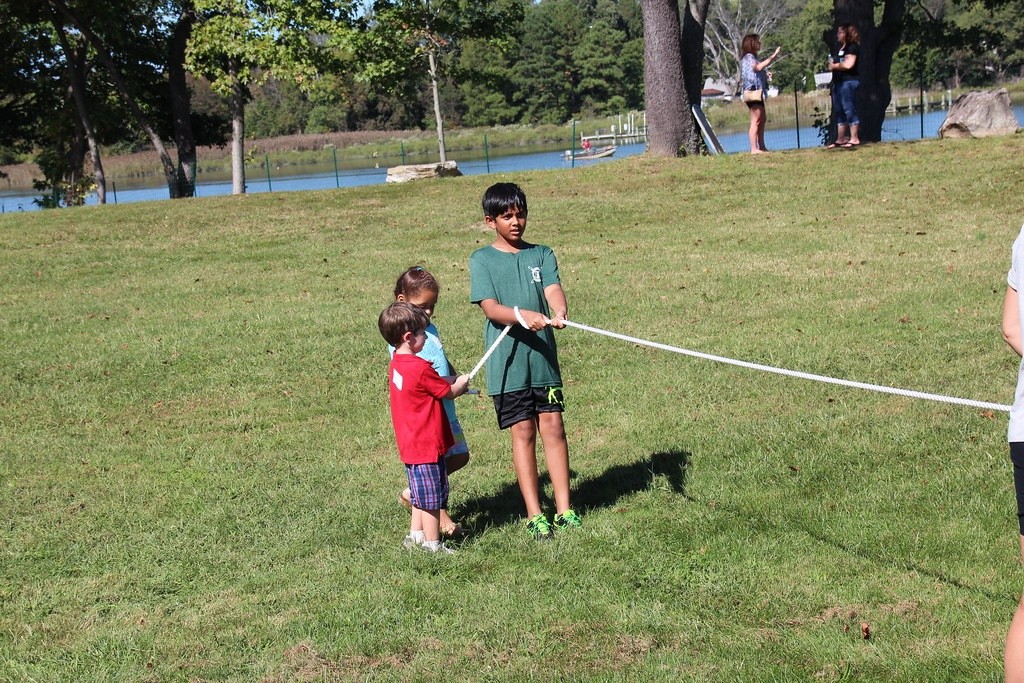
[750,149,766,154]
[404,533,424,549]
[422,540,455,554]
[440,522,464,538]
[398,487,412,514]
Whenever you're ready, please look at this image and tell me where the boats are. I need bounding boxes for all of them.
[560,144,616,161]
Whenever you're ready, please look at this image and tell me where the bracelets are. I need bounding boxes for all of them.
[768,57,773,61]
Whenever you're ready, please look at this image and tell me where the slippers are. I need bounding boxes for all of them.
[840,142,860,148]
[826,142,848,149]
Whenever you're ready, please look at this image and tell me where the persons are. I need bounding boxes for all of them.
[469,182,583,541]
[583,138,591,150]
[825,21,861,149]
[740,34,781,154]
[378,265,471,555]
[1003,223,1024,683]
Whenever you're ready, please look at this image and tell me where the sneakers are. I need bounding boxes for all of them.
[525,513,554,543]
[553,509,583,531]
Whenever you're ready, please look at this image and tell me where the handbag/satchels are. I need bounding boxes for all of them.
[743,88,762,102]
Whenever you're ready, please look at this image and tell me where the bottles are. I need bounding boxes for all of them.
[827,54,834,65]
